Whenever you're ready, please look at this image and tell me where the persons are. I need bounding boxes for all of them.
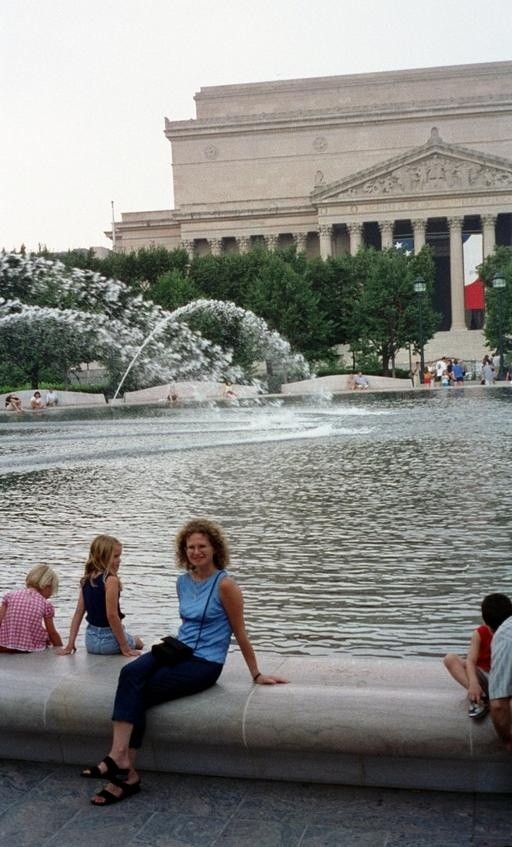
[442,593,511,718]
[55,535,144,657]
[347,373,357,391]
[411,354,496,388]
[1,564,65,652]
[488,614,511,745]
[355,371,369,390]
[5,391,25,412]
[31,391,47,409]
[79,516,289,806]
[45,386,59,406]
[223,378,240,398]
[504,370,512,385]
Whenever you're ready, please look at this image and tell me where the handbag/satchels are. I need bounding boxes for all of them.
[151,635,194,665]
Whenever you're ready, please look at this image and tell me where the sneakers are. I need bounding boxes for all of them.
[467,691,490,718]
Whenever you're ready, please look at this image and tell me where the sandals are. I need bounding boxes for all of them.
[89,774,142,806]
[79,756,130,781]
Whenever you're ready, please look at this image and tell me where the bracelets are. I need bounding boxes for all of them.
[253,669,262,679]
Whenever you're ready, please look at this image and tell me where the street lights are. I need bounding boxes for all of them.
[412,274,428,384]
[491,272,507,382]
